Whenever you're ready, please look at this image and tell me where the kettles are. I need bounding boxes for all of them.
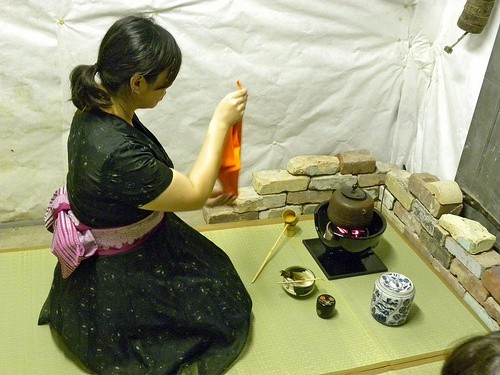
[327,184,374,229]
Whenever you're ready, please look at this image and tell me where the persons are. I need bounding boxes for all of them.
[38,15,253,375]
[441,331,500,375]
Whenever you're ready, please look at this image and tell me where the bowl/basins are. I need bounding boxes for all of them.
[282,266,315,297]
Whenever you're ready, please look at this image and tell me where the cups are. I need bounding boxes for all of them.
[316,294,336,318]
